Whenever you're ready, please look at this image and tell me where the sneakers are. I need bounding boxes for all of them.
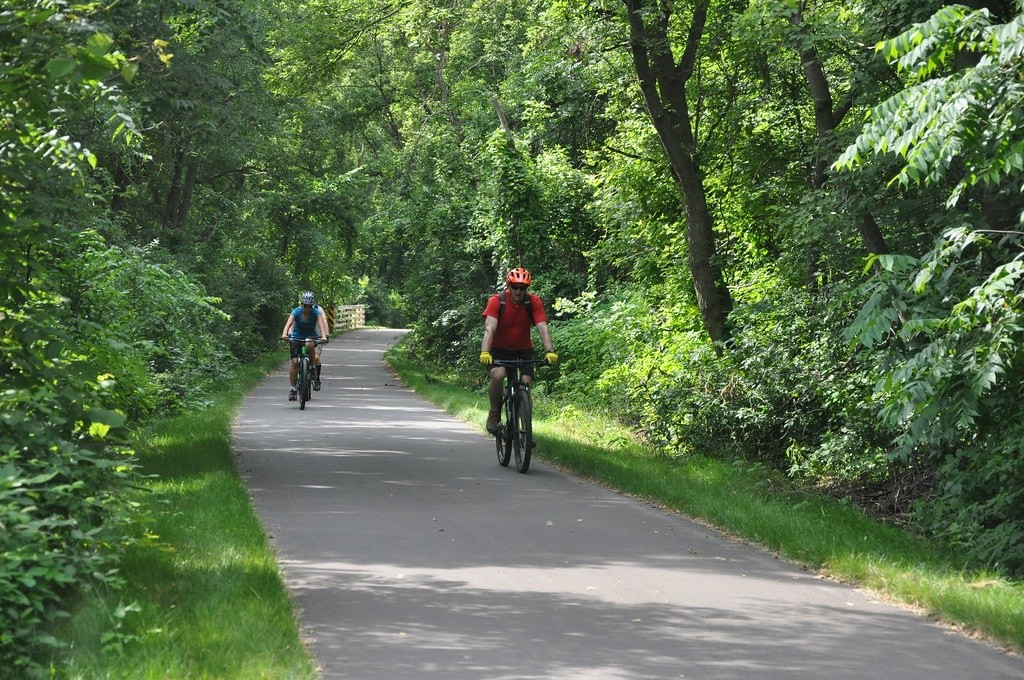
[289,391,298,401]
[486,411,501,433]
[520,436,536,448]
[314,380,322,390]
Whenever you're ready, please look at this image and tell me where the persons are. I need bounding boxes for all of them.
[281,291,327,402]
[288,292,330,392]
[478,267,559,451]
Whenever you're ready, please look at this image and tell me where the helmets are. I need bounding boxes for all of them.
[299,290,315,313]
[505,267,531,286]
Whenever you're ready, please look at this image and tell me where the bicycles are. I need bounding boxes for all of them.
[481,357,551,473]
[278,335,328,409]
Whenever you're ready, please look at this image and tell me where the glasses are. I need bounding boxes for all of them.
[302,304,313,309]
[510,285,527,290]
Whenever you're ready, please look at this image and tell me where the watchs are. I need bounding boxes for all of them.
[545,349,555,353]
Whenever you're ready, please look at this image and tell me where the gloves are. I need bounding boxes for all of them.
[480,352,492,364]
[544,349,558,365]
